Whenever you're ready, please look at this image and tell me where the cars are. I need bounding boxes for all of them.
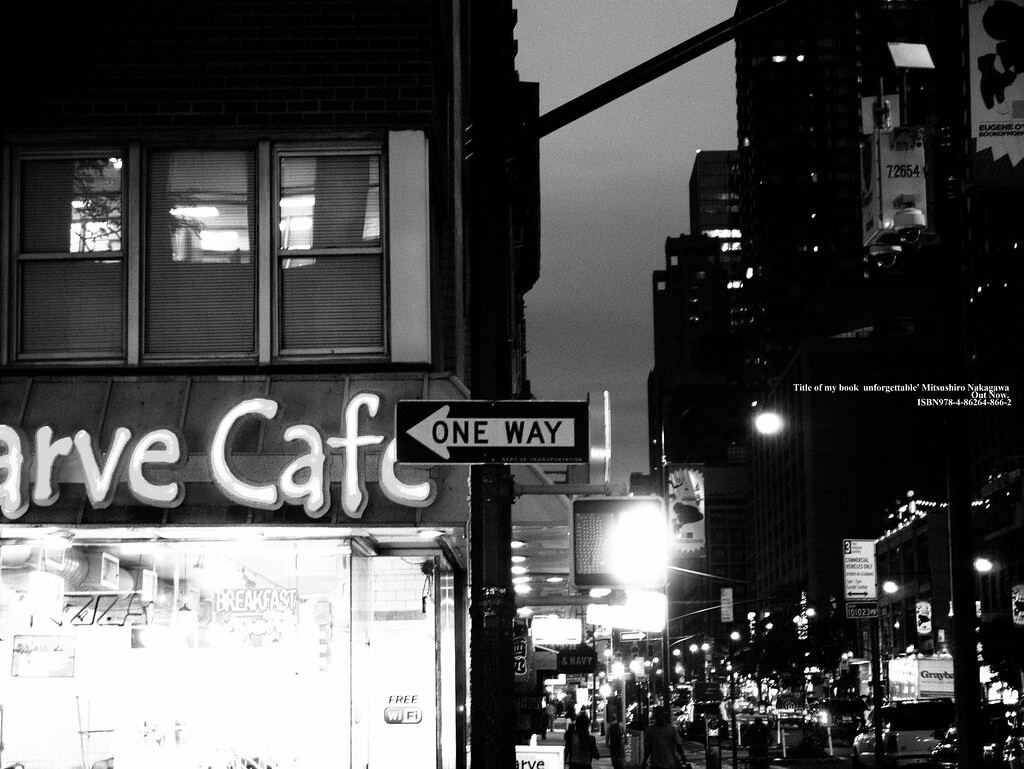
[724,699,752,714]
[674,701,730,737]
[741,700,772,714]
[801,699,864,746]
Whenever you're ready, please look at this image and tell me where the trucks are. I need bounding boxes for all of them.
[830,657,954,710]
[766,706,805,729]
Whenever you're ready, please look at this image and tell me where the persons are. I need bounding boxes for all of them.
[604,713,628,769]
[546,702,556,731]
[642,707,687,769]
[578,706,587,715]
[741,716,774,769]
[563,715,600,769]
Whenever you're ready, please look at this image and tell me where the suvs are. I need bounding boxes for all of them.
[853,696,955,769]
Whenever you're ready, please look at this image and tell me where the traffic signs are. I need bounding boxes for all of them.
[393,399,590,465]
[620,632,648,644]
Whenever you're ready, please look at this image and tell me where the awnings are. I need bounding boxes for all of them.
[511,464,573,598]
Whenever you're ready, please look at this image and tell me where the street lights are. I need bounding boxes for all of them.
[591,636,612,732]
[729,631,741,769]
[657,335,786,708]
